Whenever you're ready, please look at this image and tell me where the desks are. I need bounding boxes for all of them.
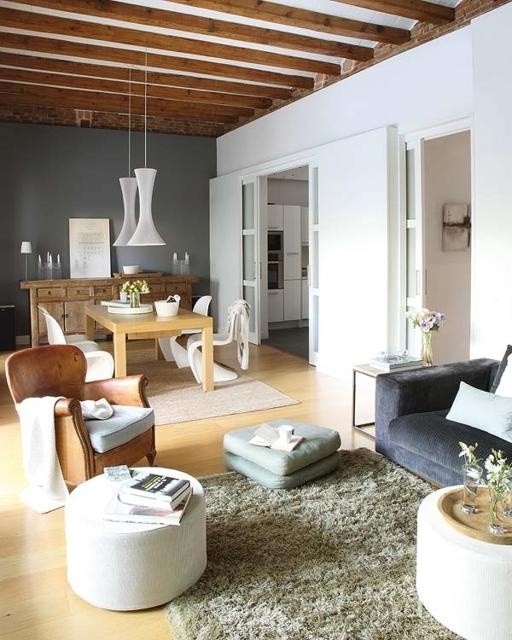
[85,302,215,393]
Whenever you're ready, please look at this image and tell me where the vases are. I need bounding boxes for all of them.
[420,333,434,367]
[130,291,141,308]
[485,491,509,537]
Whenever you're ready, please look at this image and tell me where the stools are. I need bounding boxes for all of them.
[414,483,512,638]
[66,466,210,614]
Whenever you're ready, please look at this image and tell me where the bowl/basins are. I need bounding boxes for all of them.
[154,299,182,318]
[122,265,141,274]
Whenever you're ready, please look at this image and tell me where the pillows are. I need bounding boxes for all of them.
[445,379,512,443]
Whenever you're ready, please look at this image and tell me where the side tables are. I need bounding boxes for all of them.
[350,363,382,441]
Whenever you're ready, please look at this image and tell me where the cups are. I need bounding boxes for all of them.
[457,463,512,535]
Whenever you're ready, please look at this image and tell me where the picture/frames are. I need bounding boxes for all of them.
[68,217,113,279]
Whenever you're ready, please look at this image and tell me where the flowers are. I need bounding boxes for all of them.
[406,307,448,364]
[454,441,511,526]
[122,279,150,307]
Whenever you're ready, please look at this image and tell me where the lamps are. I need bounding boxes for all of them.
[110,49,172,248]
[21,240,33,280]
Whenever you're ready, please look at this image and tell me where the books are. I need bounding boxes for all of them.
[247,423,304,453]
[102,464,133,482]
[369,355,424,369]
[129,472,190,501]
[116,475,191,510]
[102,487,194,526]
[368,362,423,374]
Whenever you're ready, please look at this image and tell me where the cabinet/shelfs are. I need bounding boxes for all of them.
[267,205,283,232]
[283,205,302,321]
[268,289,283,323]
[17,270,199,348]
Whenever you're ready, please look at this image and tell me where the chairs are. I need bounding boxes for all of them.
[39,307,114,384]
[157,295,246,385]
[4,345,157,490]
[376,358,512,493]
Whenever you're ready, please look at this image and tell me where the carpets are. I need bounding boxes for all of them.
[109,347,302,425]
[165,446,465,640]
